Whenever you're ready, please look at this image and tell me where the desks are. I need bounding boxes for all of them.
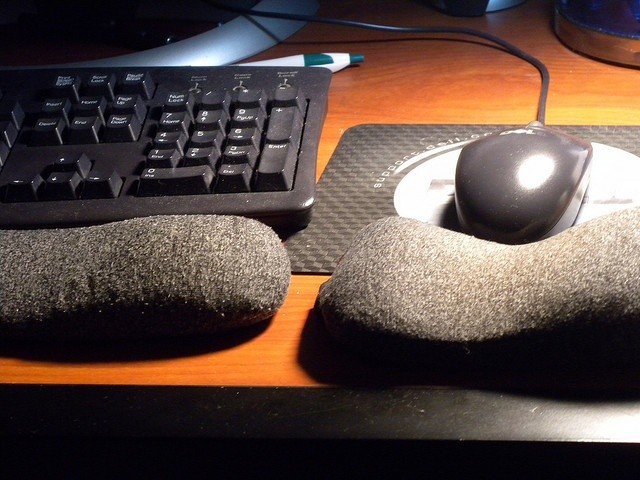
[1,0,640,469]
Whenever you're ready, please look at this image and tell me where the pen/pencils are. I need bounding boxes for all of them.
[230,52,364,74]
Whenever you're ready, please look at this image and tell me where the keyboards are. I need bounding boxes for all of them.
[1,67,332,228]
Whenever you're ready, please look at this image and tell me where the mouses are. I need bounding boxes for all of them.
[455,121,593,244]
